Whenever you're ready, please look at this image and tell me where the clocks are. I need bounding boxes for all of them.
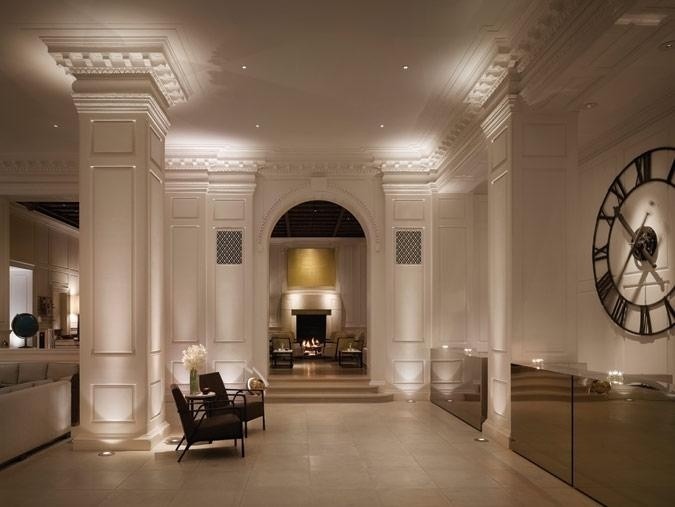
[591,145,674,338]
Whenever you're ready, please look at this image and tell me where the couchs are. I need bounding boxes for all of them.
[0,361,81,468]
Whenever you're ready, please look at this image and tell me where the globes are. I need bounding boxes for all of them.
[11,312,39,348]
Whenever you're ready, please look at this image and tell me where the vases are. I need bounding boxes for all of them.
[189,368,199,394]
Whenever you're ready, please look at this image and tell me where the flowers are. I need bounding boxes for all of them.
[183,342,206,394]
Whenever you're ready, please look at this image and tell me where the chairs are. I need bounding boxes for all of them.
[167,371,264,462]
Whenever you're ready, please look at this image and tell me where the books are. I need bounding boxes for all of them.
[32,327,55,350]
